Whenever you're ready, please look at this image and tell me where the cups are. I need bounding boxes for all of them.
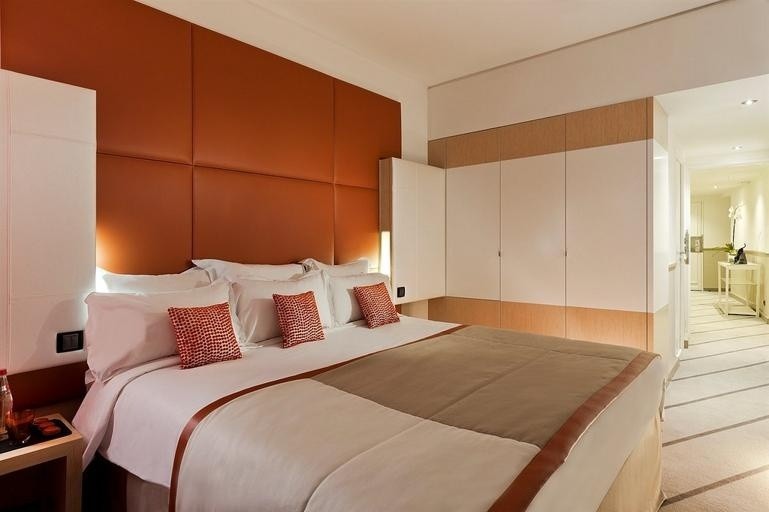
[4,407,36,445]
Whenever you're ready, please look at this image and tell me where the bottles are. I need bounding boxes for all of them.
[0,367,14,440]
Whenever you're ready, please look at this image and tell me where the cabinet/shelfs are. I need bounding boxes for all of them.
[718,261,759,317]
[428,97,668,389]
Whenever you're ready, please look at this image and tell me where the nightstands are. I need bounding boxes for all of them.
[0,414,87,512]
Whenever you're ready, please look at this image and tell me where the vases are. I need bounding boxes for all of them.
[728,253,736,263]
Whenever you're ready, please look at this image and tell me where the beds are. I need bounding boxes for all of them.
[75,258,672,512]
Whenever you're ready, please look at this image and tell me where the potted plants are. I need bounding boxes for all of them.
[712,201,746,257]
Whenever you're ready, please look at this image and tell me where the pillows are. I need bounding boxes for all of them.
[188,258,307,280]
[74,276,262,386]
[350,282,401,329]
[270,292,325,349]
[95,266,211,292]
[220,271,337,345]
[320,273,397,332]
[295,256,370,271]
[167,304,241,368]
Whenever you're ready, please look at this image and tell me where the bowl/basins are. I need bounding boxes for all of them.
[33,417,62,437]
